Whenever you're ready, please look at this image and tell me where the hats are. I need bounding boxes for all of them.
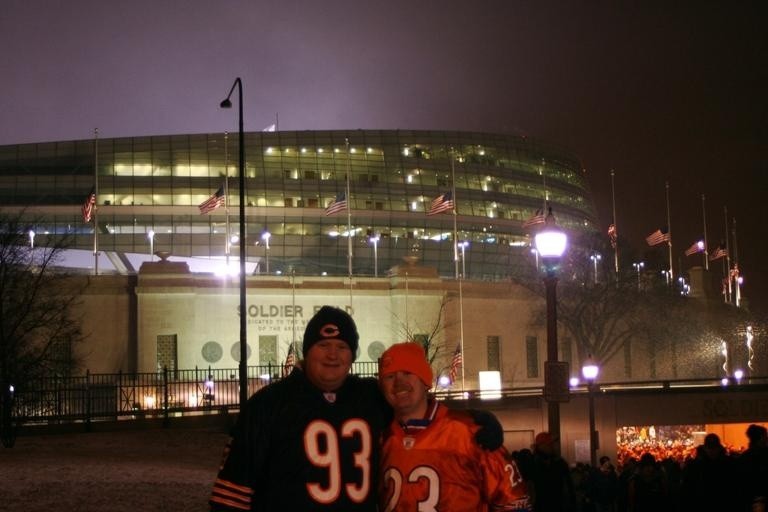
[304,305,358,361]
[536,432,554,444]
[379,343,432,386]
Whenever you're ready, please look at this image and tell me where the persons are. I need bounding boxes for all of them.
[203,373,215,407]
[370,338,533,511]
[204,302,505,510]
[507,423,768,512]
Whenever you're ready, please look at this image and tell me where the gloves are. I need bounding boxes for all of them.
[475,414,503,450]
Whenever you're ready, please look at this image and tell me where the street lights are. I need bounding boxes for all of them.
[27,228,37,272]
[220,74,248,417]
[632,261,645,290]
[261,231,271,276]
[458,241,471,278]
[590,253,601,283]
[529,248,540,271]
[660,269,692,298]
[735,276,745,304]
[368,236,379,279]
[226,235,241,266]
[147,230,155,263]
[580,360,600,467]
[527,205,575,465]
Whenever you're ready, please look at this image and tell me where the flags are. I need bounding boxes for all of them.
[683,238,704,257]
[707,240,727,262]
[80,183,95,224]
[424,190,454,217]
[518,203,544,229]
[197,182,225,217]
[283,342,293,378]
[718,263,738,290]
[323,190,347,217]
[447,342,463,386]
[644,223,671,247]
[605,223,617,249]
